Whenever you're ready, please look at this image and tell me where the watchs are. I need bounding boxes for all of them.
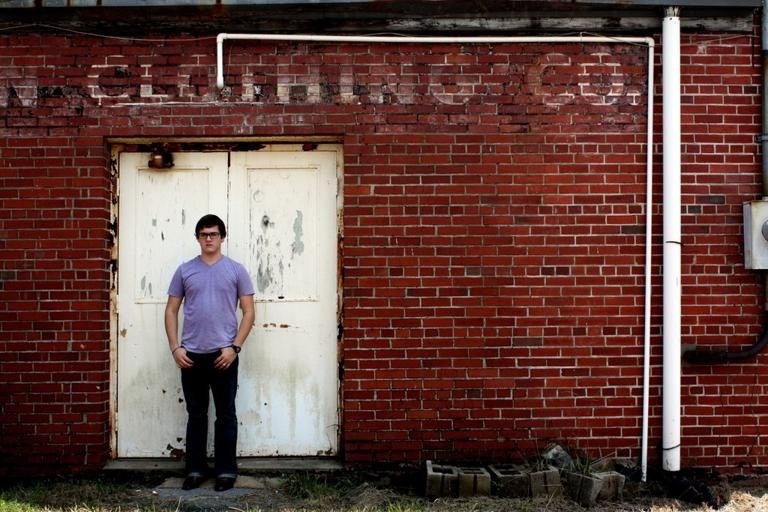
[232,345,241,354]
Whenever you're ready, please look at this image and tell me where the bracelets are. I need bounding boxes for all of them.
[170,346,180,354]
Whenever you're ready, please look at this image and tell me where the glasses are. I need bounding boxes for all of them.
[198,232,219,239]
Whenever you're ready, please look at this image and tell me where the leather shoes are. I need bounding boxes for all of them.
[182,476,208,490]
[214,477,235,491]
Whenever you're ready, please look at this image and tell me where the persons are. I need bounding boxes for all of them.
[163,214,254,490]
